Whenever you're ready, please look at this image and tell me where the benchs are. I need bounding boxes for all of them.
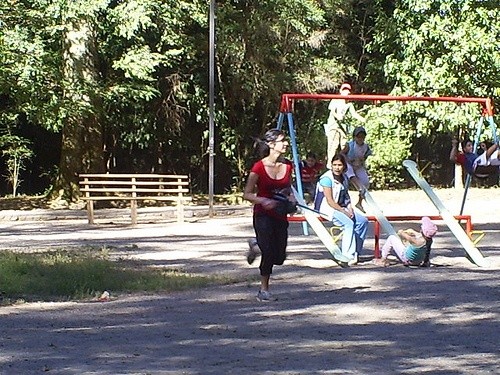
[78,173,191,225]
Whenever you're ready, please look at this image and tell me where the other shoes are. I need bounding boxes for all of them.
[373,259,390,267]
[355,203,366,213]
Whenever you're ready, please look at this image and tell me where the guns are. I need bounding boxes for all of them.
[272,193,329,219]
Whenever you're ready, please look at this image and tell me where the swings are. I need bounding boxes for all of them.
[431,102,500,179]
[297,99,355,200]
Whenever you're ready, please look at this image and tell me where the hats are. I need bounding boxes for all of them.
[421,217,437,238]
[353,127,366,136]
[341,84,351,90]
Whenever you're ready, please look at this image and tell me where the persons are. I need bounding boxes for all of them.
[294,83,371,267]
[243,129,293,302]
[450,139,500,179]
[373,216,437,267]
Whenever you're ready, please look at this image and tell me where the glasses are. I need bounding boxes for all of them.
[274,137,286,142]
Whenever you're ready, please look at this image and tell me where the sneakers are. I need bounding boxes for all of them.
[247,239,257,264]
[256,290,278,303]
